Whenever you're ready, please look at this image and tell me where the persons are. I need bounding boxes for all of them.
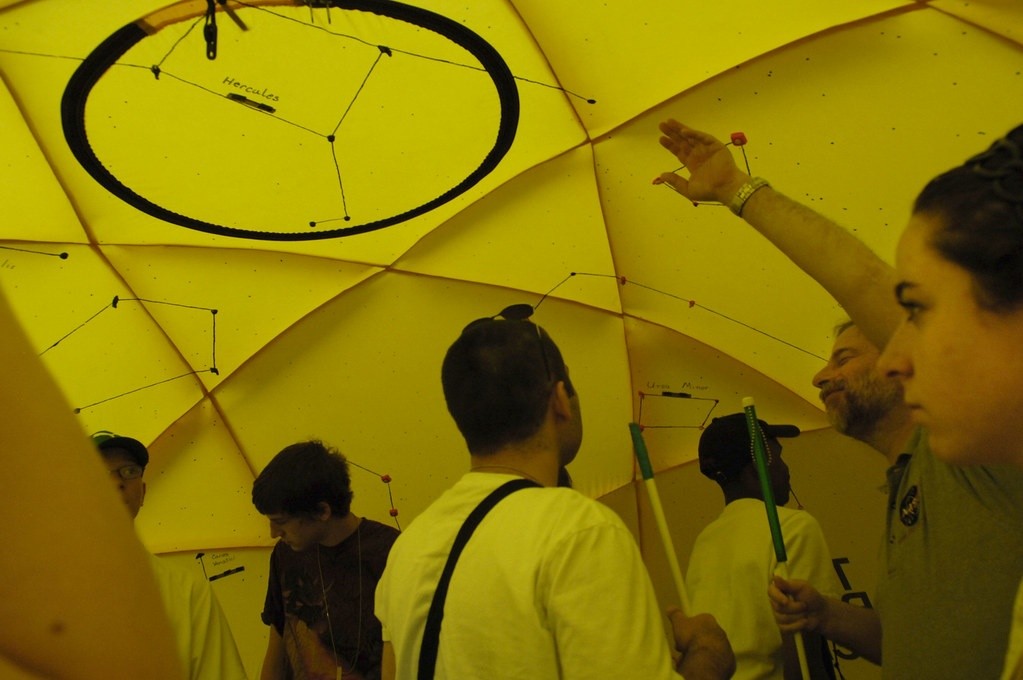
[874,119,1023,680]
[681,411,844,679]
[0,290,190,680]
[252,442,403,680]
[651,117,1022,679]
[85,424,249,679]
[372,320,737,679]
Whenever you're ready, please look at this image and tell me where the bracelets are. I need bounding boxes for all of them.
[729,175,771,218]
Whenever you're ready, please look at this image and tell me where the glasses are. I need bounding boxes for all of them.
[460,303,551,381]
[109,465,143,480]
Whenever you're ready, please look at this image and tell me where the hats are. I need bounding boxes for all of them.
[88,430,149,470]
[698,411,800,480]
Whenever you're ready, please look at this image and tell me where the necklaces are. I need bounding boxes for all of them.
[315,514,363,674]
